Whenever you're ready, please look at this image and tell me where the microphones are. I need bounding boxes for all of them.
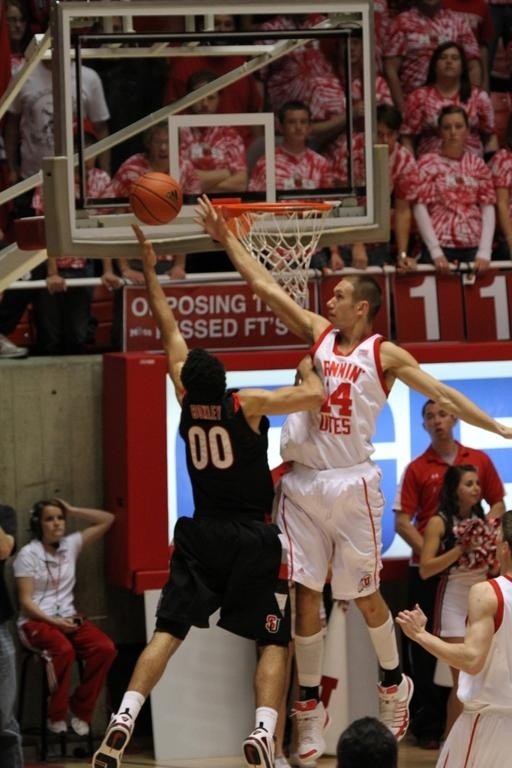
[37,539,60,549]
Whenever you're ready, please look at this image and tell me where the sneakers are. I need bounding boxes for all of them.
[47,720,68,735]
[70,718,91,736]
[243,720,276,768]
[376,674,414,743]
[291,700,331,763]
[93,708,135,768]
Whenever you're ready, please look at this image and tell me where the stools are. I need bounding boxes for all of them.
[19,640,95,761]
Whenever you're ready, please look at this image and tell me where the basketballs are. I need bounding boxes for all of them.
[130,172,183,226]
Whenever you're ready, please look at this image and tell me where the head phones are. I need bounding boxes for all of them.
[31,510,42,537]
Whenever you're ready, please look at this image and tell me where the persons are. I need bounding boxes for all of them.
[92,224,329,767]
[2,1,512,360]
[1,499,117,768]
[255,462,349,767]
[193,194,512,764]
[393,399,512,768]
[336,718,397,768]
